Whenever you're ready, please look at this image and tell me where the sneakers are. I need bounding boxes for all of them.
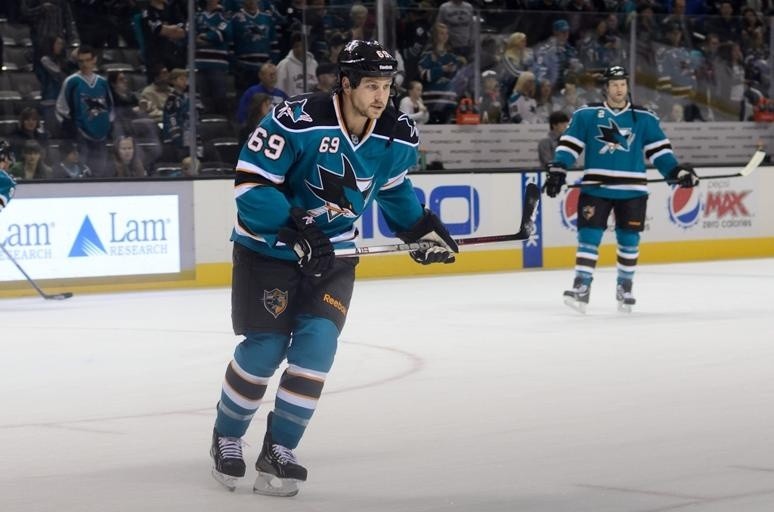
[210,427,245,477]
[563,277,589,302]
[256,411,307,480]
[616,279,636,304]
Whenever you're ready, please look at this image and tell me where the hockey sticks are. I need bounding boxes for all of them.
[0,244,73,300]
[335,183,540,257]
[559,148,766,190]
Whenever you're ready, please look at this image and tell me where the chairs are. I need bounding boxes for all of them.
[0,0,328,176]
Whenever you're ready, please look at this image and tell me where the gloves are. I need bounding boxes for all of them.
[395,209,459,265]
[674,162,699,188]
[542,167,566,198]
[273,206,335,280]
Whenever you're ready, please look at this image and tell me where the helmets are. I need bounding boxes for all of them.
[336,39,397,85]
[603,65,630,82]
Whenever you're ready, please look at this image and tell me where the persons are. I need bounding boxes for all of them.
[0,165,19,215]
[0,0,378,180]
[541,64,706,309]
[201,34,462,485]
[539,111,569,170]
[395,0,774,124]
